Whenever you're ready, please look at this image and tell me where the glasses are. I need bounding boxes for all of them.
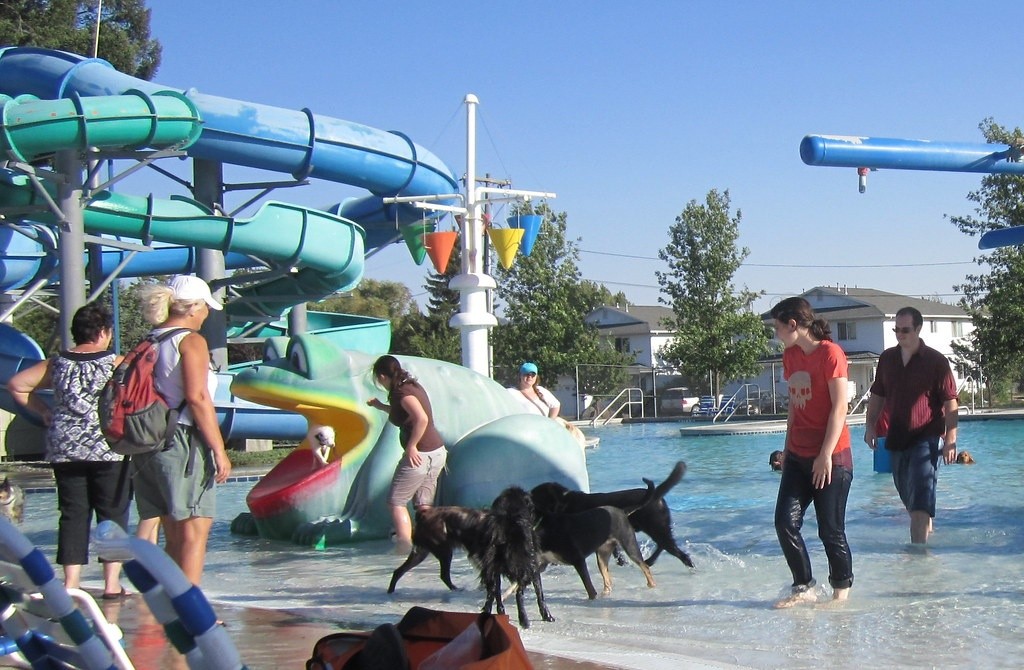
[892,327,914,333]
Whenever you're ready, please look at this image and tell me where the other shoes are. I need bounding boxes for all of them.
[102,586,131,599]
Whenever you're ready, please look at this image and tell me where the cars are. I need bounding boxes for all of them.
[660,387,701,416]
[735,390,790,415]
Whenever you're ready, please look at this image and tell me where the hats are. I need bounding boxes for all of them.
[520,362,538,375]
[168,276,224,311]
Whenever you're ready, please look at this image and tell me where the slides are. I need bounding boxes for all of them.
[0,46,590,550]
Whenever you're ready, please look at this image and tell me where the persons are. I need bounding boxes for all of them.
[507,362,562,422]
[862,306,958,544]
[367,356,445,556]
[129,275,232,628]
[8,305,133,600]
[773,296,855,612]
[768,450,783,471]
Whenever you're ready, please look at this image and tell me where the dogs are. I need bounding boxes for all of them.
[387,459,694,629]
[307,423,337,472]
[0,476,25,524]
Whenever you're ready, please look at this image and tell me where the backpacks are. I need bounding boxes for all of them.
[98,328,198,455]
